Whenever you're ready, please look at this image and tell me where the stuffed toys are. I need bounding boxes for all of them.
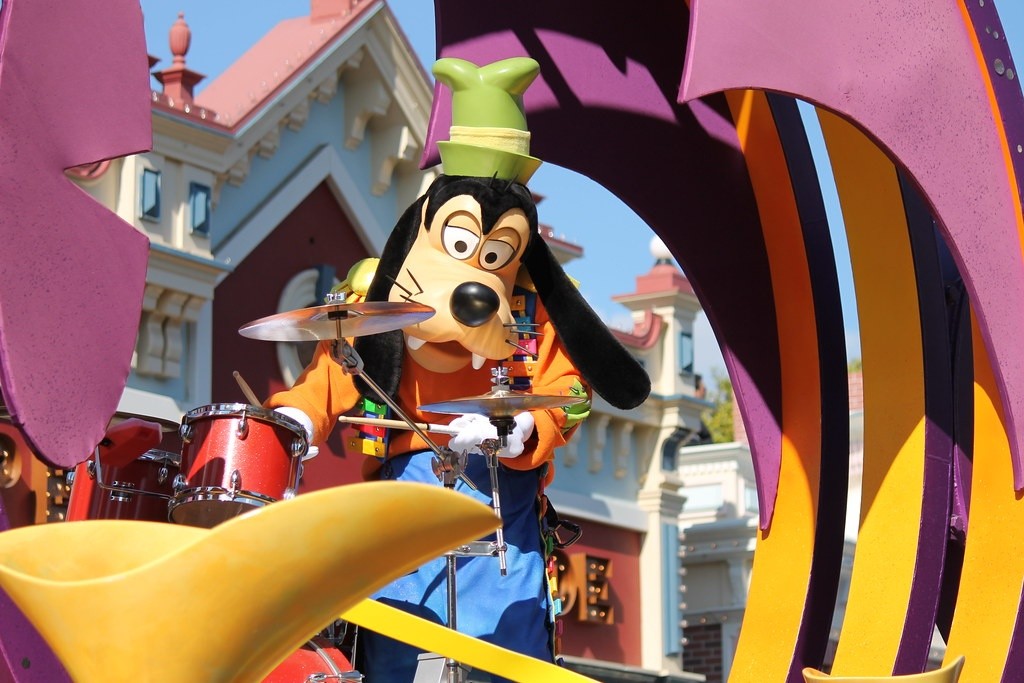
[260,59,652,683]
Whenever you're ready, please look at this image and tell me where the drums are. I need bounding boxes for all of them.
[168,399,311,530]
[259,630,367,683]
[60,409,183,524]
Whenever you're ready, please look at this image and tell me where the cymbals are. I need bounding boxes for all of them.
[417,388,590,419]
[237,299,437,343]
